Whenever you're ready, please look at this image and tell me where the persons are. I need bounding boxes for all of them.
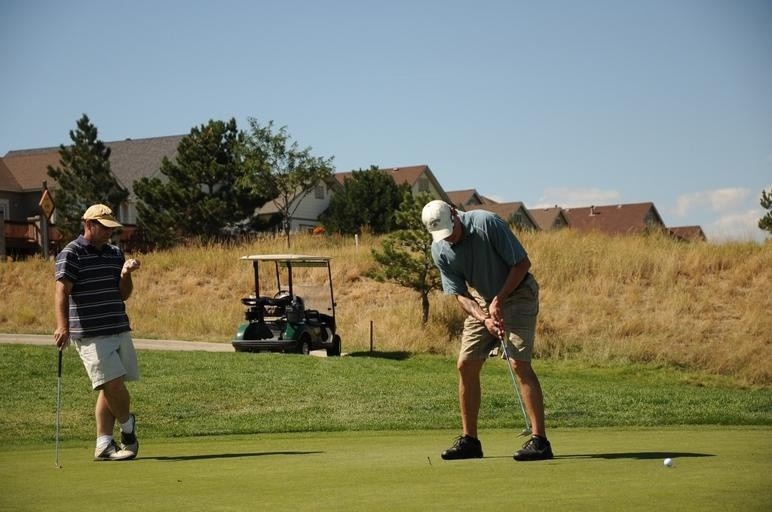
[52,203,140,461]
[420,199,555,462]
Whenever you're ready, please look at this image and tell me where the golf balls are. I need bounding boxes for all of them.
[664,458,672,466]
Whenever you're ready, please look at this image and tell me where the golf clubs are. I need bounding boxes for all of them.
[498,335,533,439]
[55,342,63,468]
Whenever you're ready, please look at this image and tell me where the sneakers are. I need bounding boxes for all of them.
[442,436,482,459]
[93,440,133,461]
[120,414,138,457]
[513,438,553,460]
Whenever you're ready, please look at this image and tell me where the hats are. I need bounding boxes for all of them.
[421,200,454,242]
[83,203,124,227]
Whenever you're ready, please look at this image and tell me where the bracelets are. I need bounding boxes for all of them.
[481,315,491,325]
